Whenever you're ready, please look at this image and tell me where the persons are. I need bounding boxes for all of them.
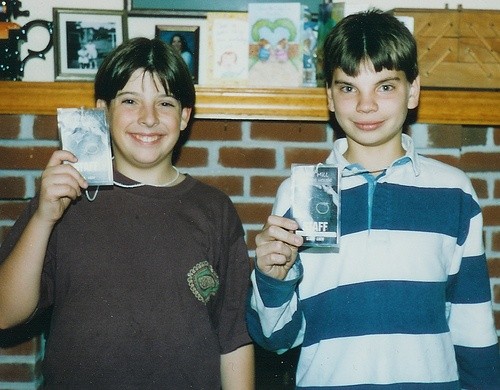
[0,37,255,390]
[170,34,196,82]
[77,39,98,68]
[247,5,499,390]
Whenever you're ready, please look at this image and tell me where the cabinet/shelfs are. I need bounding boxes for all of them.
[385,8,500,90]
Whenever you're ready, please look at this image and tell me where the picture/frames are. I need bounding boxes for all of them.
[52,7,129,81]
[154,25,201,84]
